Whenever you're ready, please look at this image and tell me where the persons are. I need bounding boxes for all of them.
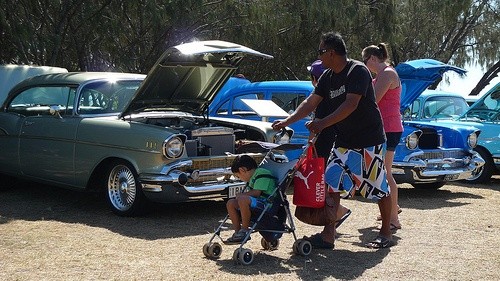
[361,42,402,231]
[304,57,352,229]
[225,155,279,241]
[272,32,393,251]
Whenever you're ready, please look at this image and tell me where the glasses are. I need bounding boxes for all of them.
[362,57,371,66]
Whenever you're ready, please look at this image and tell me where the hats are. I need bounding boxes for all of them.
[307,59,327,76]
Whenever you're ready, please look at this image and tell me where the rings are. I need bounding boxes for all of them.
[312,129,316,132]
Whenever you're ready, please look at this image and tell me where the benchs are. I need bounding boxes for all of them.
[6,105,104,117]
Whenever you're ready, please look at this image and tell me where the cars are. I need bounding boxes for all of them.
[1,39,295,218]
[404,94,481,126]
[206,58,484,194]
[451,83,500,183]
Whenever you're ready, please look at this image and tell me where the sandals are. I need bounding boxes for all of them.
[365,236,394,249]
[304,232,337,249]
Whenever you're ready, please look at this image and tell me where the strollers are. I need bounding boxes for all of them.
[202,123,313,265]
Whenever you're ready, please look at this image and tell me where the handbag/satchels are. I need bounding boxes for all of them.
[293,143,326,209]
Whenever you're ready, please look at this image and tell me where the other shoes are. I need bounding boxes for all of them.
[227,230,251,243]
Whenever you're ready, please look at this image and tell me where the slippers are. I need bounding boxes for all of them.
[377,206,403,220]
[336,211,352,228]
[388,223,403,230]
